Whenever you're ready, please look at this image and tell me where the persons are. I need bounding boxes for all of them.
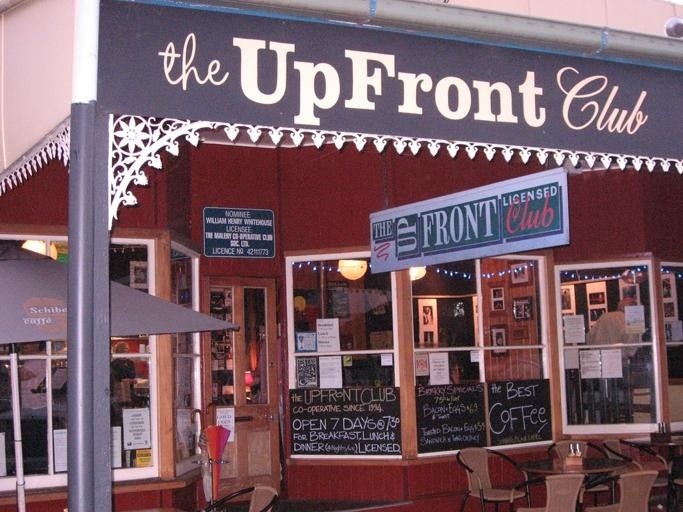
[581,296,641,416]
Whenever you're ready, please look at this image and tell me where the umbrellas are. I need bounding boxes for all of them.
[0,241,242,512]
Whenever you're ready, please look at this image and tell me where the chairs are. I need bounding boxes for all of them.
[454,435,683,512]
[202,484,278,512]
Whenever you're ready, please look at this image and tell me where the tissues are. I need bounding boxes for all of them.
[562,443,583,466]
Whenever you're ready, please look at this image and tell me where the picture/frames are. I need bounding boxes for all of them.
[490,323,509,357]
[507,260,534,323]
